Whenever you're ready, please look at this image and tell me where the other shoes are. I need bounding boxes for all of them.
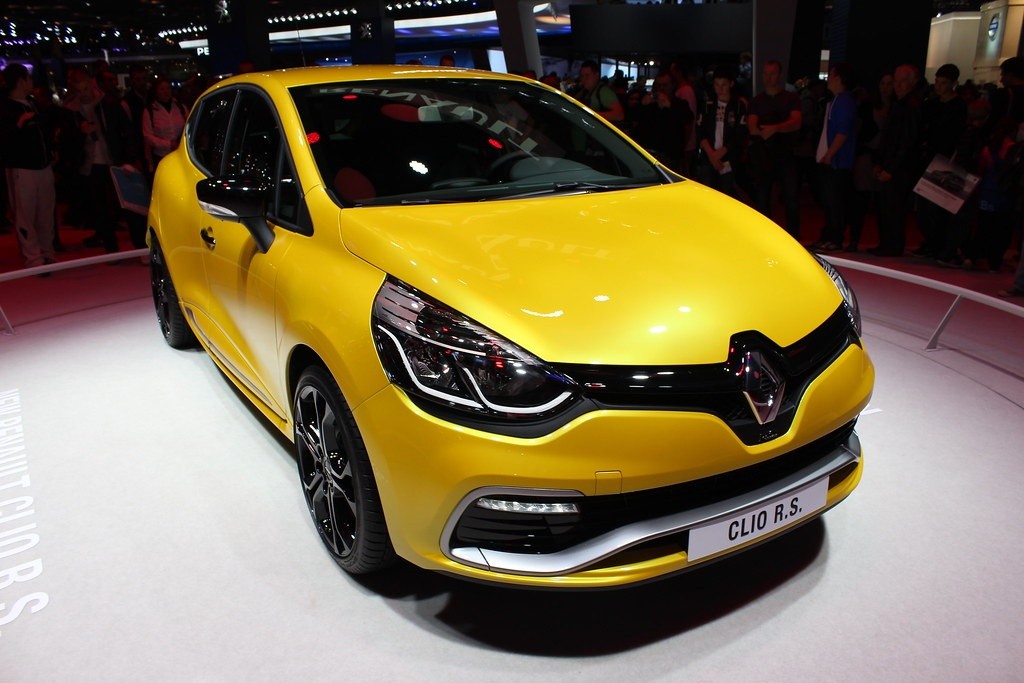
[868,248,902,256]
[106,250,119,265]
[33,272,50,277]
[85,236,107,248]
[140,254,149,266]
[808,240,844,253]
[911,248,935,257]
[937,257,966,268]
[976,258,992,272]
[848,243,857,253]
[54,242,64,252]
[44,257,56,265]
[999,286,1023,297]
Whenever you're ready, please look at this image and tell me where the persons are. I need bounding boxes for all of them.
[410,54,1023,297]
[0,57,257,281]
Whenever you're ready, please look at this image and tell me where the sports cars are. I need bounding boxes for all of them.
[144,65,874,597]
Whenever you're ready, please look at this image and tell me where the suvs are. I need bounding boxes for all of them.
[930,169,966,193]
